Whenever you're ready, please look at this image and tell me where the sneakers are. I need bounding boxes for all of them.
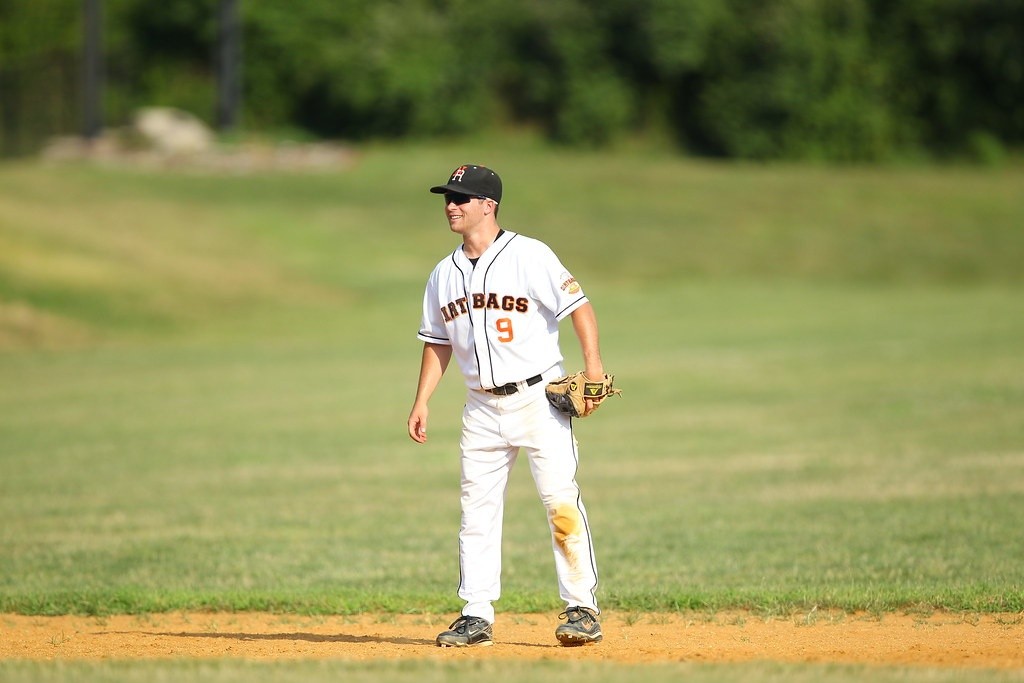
[555,606,603,647]
[436,615,494,650]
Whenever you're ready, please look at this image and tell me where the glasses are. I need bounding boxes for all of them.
[444,193,499,206]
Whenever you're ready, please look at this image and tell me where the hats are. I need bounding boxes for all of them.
[430,164,502,204]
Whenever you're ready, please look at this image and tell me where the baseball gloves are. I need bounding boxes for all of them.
[544,369,624,419]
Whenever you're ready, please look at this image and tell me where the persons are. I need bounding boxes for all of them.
[408,165,606,648]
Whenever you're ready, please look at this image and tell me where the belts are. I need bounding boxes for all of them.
[485,373,543,395]
